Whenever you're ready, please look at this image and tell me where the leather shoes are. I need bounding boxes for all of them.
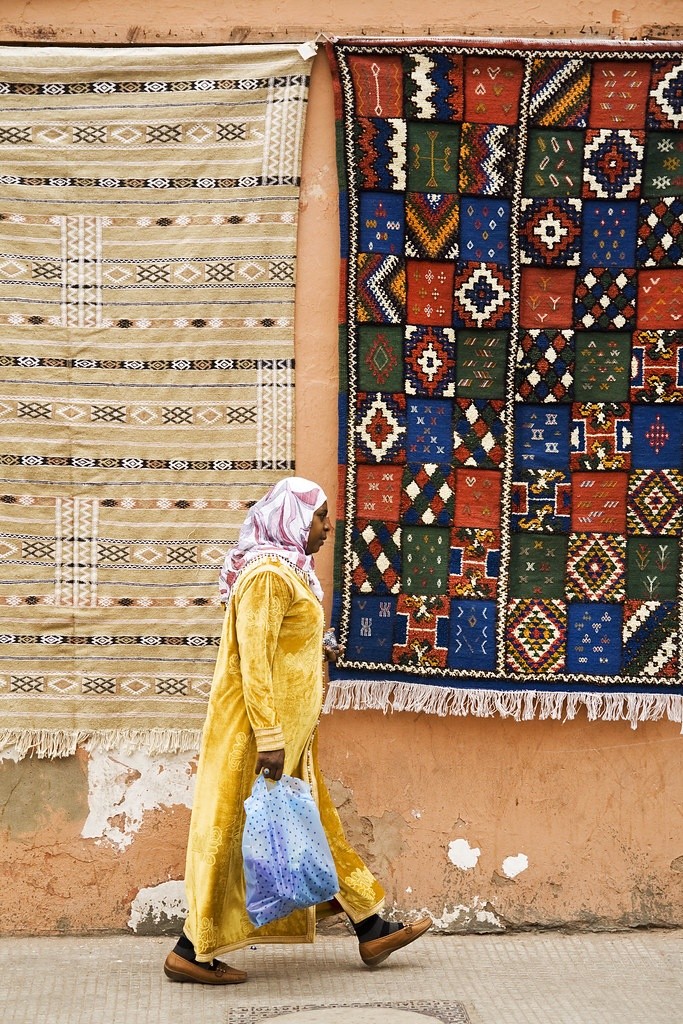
[164,951,248,985]
[359,916,433,966]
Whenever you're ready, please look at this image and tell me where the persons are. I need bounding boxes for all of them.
[163,476,434,986]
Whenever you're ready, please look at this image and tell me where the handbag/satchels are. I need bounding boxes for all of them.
[241,772,340,928]
